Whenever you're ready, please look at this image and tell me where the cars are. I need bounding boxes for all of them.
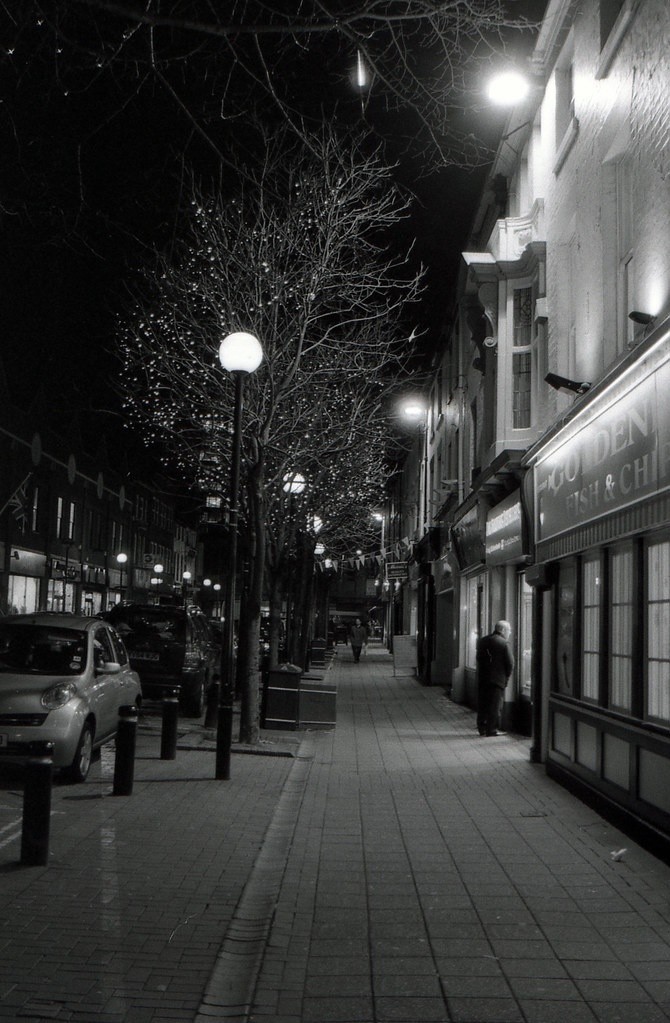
[1,610,142,785]
[209,615,293,675]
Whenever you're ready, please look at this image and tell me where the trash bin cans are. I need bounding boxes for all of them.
[310,638,325,667]
[262,663,302,730]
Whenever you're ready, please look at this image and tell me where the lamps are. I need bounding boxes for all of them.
[403,406,428,418]
[629,311,658,324]
[486,71,545,108]
[544,372,591,401]
[429,479,457,505]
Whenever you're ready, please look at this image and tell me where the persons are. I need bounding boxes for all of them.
[476,620,515,737]
[328,615,341,632]
[367,619,381,637]
[348,619,367,662]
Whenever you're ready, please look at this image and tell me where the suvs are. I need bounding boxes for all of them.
[106,599,225,719]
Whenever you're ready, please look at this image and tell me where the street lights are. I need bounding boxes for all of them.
[116,553,127,602]
[214,584,221,621]
[213,332,264,782]
[282,472,306,663]
[182,571,192,607]
[154,564,163,605]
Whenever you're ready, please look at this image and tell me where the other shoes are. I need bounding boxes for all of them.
[486,733,507,737]
